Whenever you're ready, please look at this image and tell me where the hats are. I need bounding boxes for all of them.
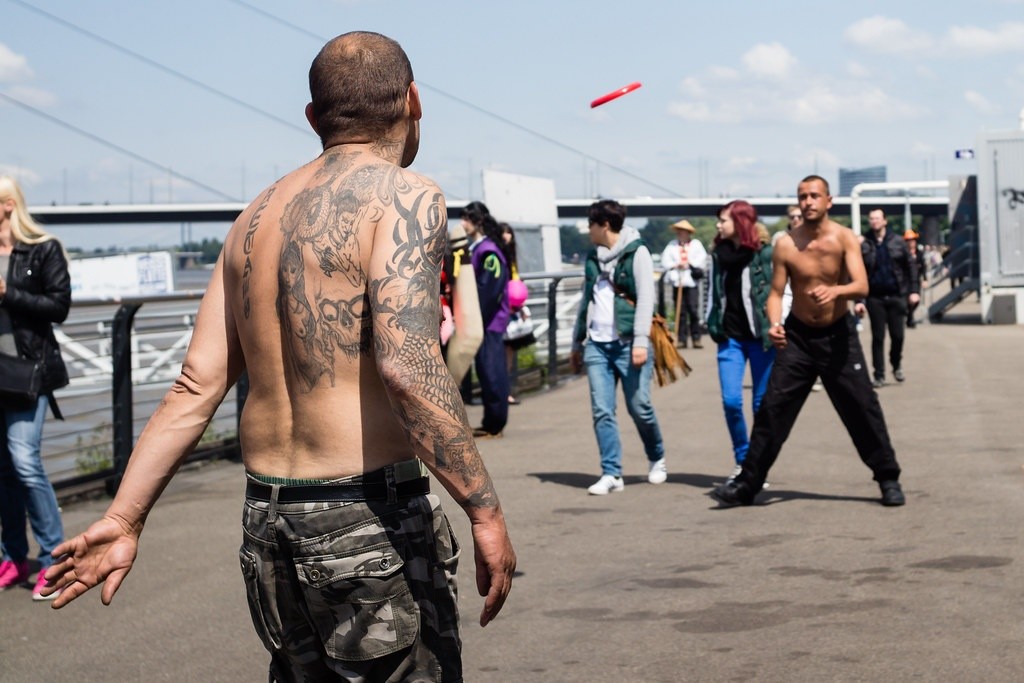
[449,227,468,249]
[903,230,919,238]
[672,220,696,233]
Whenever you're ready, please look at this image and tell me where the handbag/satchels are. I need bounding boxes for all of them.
[0,352,38,402]
[652,318,692,387]
[501,307,533,340]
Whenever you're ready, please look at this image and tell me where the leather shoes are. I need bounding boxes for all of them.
[711,483,754,505]
[881,482,904,505]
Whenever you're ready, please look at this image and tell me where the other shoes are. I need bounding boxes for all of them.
[693,341,703,348]
[677,342,686,348]
[873,379,882,387]
[507,399,520,404]
[473,430,502,438]
[894,370,904,381]
[726,466,742,485]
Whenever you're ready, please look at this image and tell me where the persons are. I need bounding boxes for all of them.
[713,174,905,507]
[707,200,778,490]
[439,201,522,440]
[42,31,518,683]
[771,207,949,388]
[661,219,707,350]
[575,200,667,496]
[0,175,72,601]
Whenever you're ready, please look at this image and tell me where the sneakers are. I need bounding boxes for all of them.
[588,475,623,494]
[0,561,30,591]
[648,459,666,484]
[32,569,60,600]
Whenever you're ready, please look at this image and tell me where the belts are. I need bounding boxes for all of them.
[246,476,430,503]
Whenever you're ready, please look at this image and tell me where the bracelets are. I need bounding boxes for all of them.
[770,322,783,327]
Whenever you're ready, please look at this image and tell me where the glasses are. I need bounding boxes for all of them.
[789,216,802,219]
